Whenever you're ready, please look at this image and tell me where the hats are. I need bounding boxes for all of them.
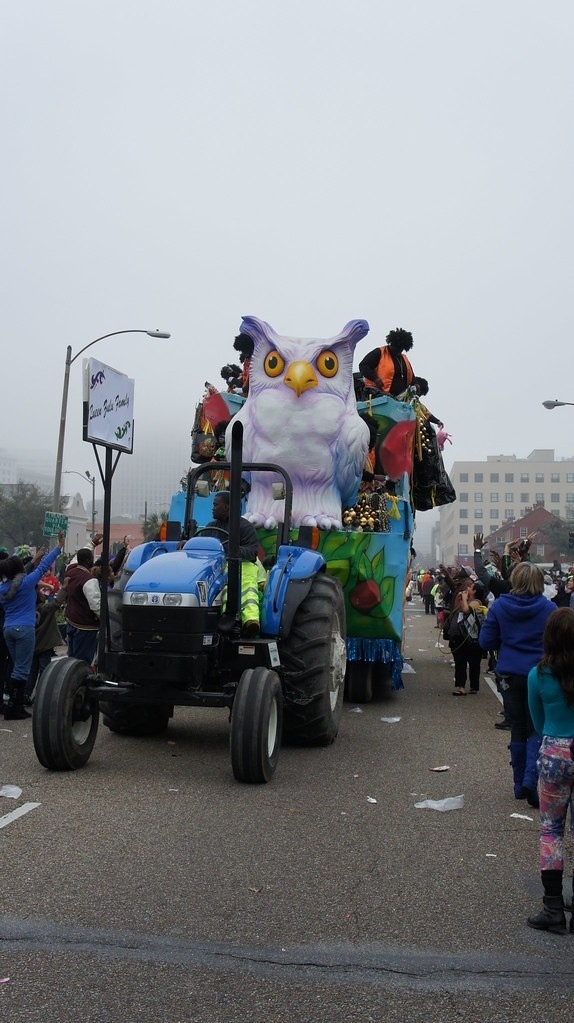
[18,546,33,562]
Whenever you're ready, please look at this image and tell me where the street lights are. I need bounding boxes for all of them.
[47,327,171,576]
[62,469,96,538]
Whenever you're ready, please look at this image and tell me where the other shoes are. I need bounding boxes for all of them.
[243,621,261,639]
[500,710,505,716]
[564,897,574,912]
[495,719,513,730]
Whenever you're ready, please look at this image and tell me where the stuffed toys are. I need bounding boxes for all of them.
[436,424,452,451]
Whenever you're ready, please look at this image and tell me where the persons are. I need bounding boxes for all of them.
[405,377,444,431]
[353,327,414,402]
[220,333,255,398]
[479,562,557,807]
[177,492,260,640]
[403,532,574,729]
[356,411,386,494]
[0,533,132,720]
[206,419,230,492]
[528,608,574,933]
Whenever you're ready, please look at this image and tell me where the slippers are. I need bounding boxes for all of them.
[469,689,477,695]
[452,688,467,695]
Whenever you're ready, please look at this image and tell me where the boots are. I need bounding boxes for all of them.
[569,868,574,934]
[507,742,527,799]
[527,870,566,931]
[521,737,539,808]
[4,679,32,720]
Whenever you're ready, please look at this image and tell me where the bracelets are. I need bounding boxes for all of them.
[503,550,510,554]
[91,541,96,547]
[475,549,482,553]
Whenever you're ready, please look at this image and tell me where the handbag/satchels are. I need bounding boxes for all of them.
[442,608,459,641]
[459,610,483,650]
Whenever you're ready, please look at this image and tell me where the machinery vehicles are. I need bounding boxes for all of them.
[31,422,413,787]
